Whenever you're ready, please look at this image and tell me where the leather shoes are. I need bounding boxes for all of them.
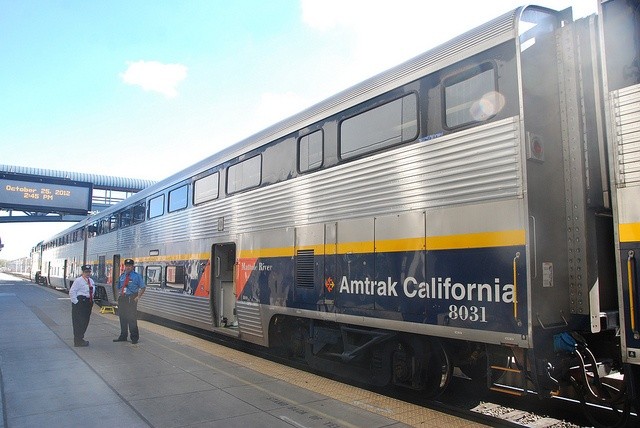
[113,336,127,342]
[132,337,138,344]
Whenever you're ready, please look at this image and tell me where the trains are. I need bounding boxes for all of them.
[30,0,640,420]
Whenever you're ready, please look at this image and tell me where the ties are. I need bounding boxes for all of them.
[87,278,92,304]
[122,274,129,298]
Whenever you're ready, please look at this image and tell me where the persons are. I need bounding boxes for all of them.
[113,260,146,344]
[69,265,96,347]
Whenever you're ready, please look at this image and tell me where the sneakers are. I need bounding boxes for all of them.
[75,338,89,347]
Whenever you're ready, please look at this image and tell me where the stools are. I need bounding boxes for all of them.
[100,305,115,314]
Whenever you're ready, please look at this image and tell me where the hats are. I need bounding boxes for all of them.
[82,265,93,271]
[125,259,134,265]
